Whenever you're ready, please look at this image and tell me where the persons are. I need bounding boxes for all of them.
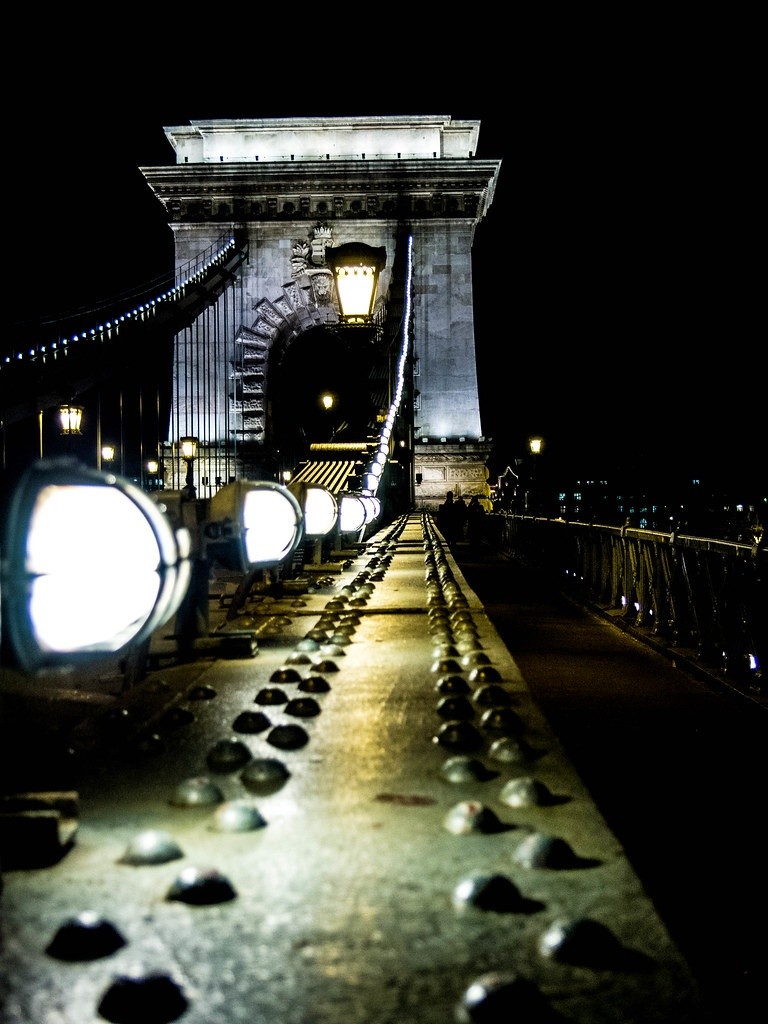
[438,492,487,545]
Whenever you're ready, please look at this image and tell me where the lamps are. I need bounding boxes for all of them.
[209,477,304,575]
[355,493,376,525]
[324,242,388,326]
[368,496,381,518]
[331,492,367,538]
[285,482,339,542]
[0,454,193,680]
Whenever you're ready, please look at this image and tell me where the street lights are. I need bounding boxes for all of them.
[180,435,201,551]
[101,442,115,472]
[324,239,386,442]
[321,390,334,441]
[59,396,82,459]
[527,435,542,515]
[146,457,159,491]
